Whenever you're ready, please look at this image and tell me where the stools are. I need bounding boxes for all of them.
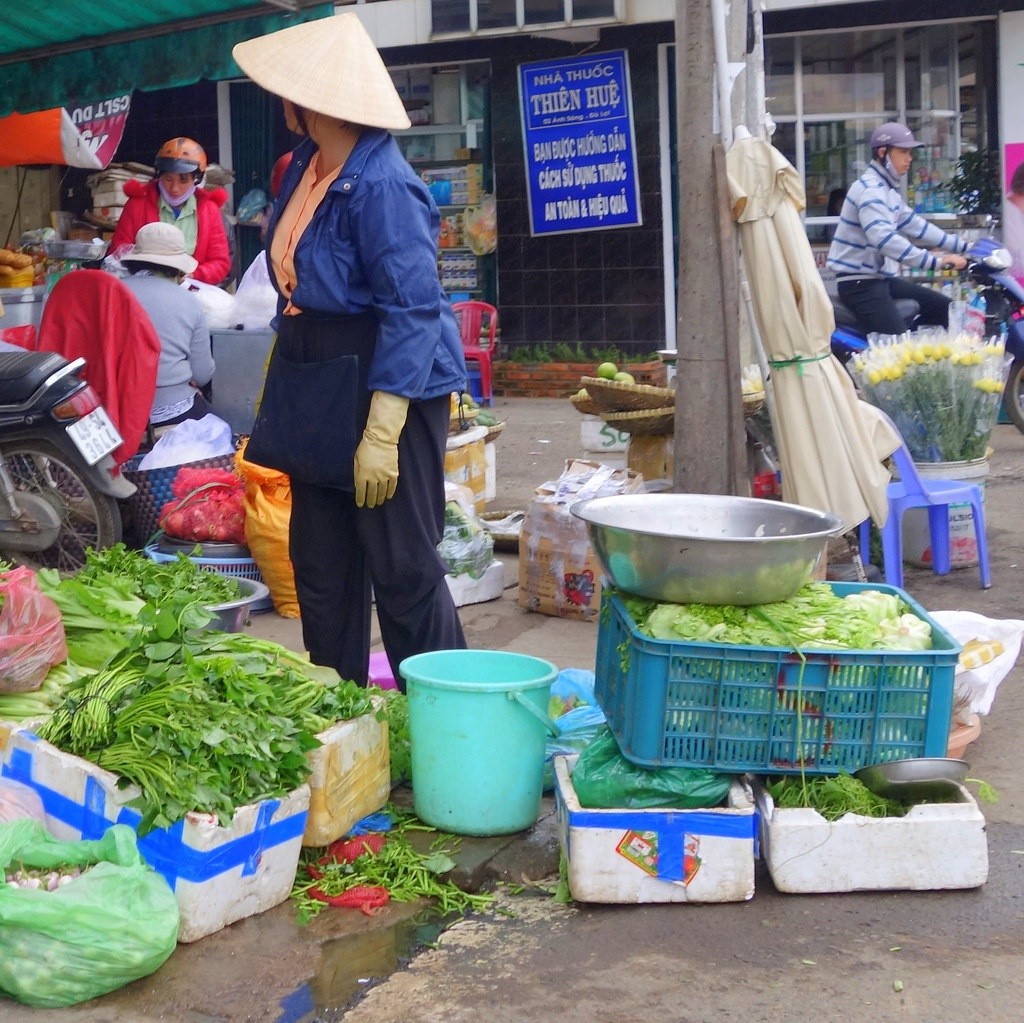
[462,361,494,408]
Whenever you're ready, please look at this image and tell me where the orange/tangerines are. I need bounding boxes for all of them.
[597,362,635,384]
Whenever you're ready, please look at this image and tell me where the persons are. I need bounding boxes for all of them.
[826,123,973,340]
[823,189,849,240]
[231,12,468,695]
[106,138,231,286]
[112,222,226,439]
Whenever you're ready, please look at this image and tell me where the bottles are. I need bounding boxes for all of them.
[892,249,961,276]
[806,141,856,206]
[439,211,465,248]
[749,443,780,501]
[908,185,961,214]
[913,142,956,162]
[909,164,956,185]
[917,281,978,304]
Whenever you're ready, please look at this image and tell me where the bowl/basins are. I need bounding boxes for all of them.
[569,493,845,605]
[368,651,399,691]
[186,575,270,638]
[947,713,981,759]
[853,758,972,798]
[43,241,109,261]
[160,536,251,558]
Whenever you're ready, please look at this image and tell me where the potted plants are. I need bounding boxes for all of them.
[941,144,1002,230]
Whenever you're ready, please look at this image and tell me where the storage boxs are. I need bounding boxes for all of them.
[44,179,136,260]
[444,559,503,608]
[590,575,963,776]
[519,458,646,622]
[298,692,390,846]
[443,426,497,505]
[441,270,481,289]
[0,718,312,944]
[750,778,988,892]
[552,755,755,905]
[440,251,481,270]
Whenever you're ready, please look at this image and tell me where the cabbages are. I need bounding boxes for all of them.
[607,579,932,765]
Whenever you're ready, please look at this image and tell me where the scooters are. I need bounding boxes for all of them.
[0,341,138,583]
[831,213,1024,436]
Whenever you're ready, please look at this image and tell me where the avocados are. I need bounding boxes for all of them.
[461,394,496,426]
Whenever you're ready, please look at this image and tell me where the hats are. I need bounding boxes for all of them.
[230,10,413,139]
[119,222,199,274]
[870,121,927,150]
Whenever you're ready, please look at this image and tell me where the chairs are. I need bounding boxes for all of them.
[851,400,991,592]
[452,301,498,399]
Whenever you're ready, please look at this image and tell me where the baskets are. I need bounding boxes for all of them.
[483,421,507,444]
[579,375,674,413]
[117,446,240,548]
[476,509,526,551]
[599,390,766,436]
[17,461,97,568]
[569,394,618,417]
[142,542,274,612]
[448,408,479,431]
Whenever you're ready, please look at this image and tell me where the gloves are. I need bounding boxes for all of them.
[353,390,412,508]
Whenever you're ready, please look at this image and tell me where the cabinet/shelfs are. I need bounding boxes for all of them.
[433,205,485,296]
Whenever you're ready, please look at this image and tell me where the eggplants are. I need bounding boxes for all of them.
[0,857,180,1007]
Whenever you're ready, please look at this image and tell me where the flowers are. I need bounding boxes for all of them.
[850,327,1015,464]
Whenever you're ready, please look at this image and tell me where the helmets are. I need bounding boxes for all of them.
[154,138,207,185]
[270,151,294,200]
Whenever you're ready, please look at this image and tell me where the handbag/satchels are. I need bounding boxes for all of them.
[243,338,364,495]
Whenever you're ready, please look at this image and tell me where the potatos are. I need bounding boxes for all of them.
[0,248,32,275]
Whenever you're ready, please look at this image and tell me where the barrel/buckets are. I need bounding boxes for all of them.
[399,650,561,837]
[887,447,993,569]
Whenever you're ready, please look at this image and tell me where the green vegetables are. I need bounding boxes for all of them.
[292,804,570,924]
[437,509,493,579]
[36,590,413,836]
[0,542,241,722]
[765,772,934,821]
